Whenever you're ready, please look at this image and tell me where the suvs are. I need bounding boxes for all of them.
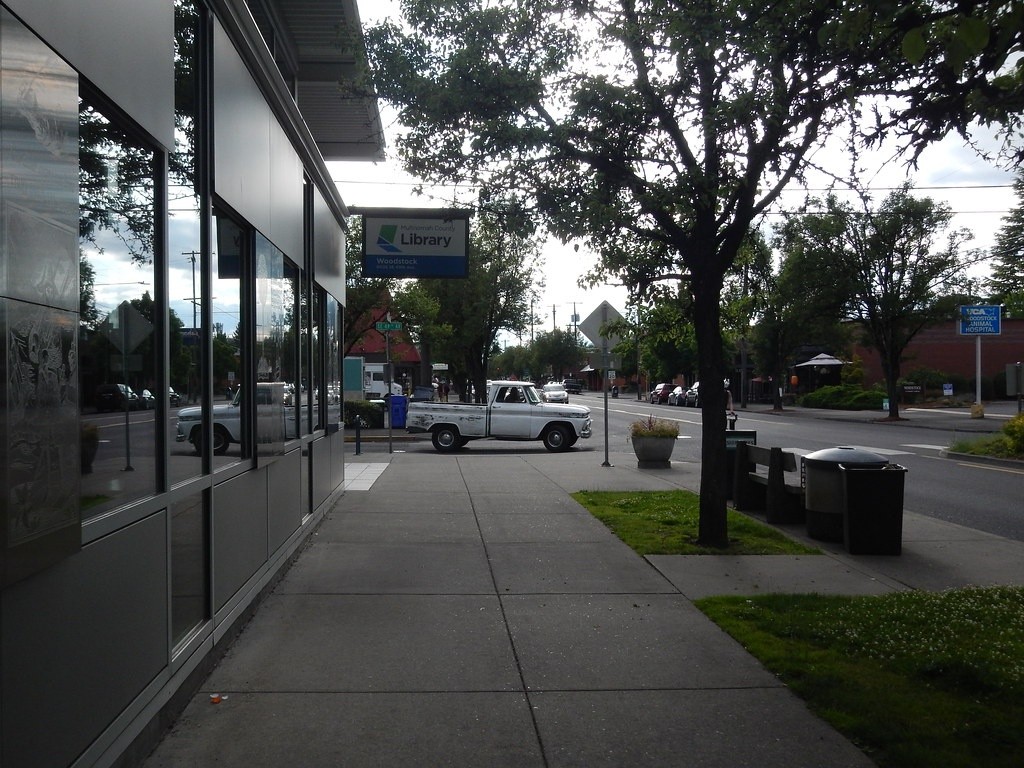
[685,381,703,408]
[650,383,678,405]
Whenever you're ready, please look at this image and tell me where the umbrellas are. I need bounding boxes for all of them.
[790,351,854,388]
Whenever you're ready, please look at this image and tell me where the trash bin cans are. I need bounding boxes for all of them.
[386,395,408,429]
[612,385,618,398]
[839,463,909,555]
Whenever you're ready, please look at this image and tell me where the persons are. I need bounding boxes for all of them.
[503,386,526,403]
[408,376,451,402]
[724,376,735,430]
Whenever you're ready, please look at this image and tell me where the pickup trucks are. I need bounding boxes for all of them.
[405,381,592,452]
[176,381,341,457]
[547,378,582,395]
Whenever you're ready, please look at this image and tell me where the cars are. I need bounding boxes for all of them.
[539,384,569,404]
[667,386,690,405]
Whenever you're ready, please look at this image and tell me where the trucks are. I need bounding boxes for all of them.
[364,361,403,400]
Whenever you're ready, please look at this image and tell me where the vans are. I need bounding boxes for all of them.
[94,381,181,413]
